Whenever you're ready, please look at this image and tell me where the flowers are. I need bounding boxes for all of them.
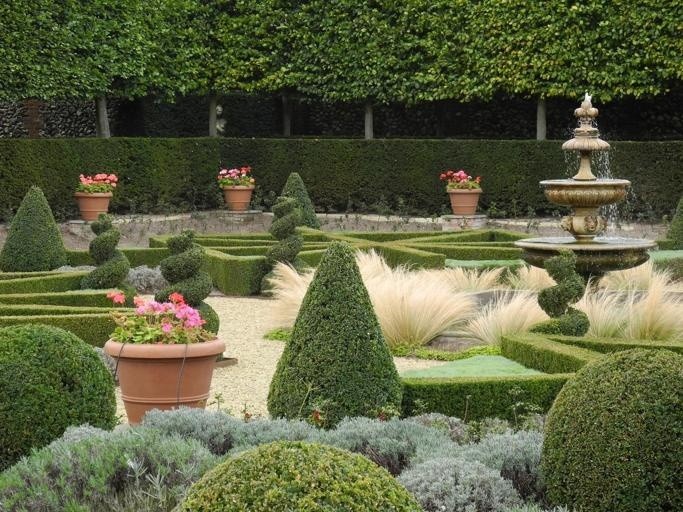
[103,288,215,345]
[216,167,255,187]
[440,170,481,190]
[75,172,117,196]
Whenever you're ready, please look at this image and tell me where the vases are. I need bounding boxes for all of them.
[222,185,255,211]
[106,336,226,425]
[74,192,113,219]
[447,188,482,215]
